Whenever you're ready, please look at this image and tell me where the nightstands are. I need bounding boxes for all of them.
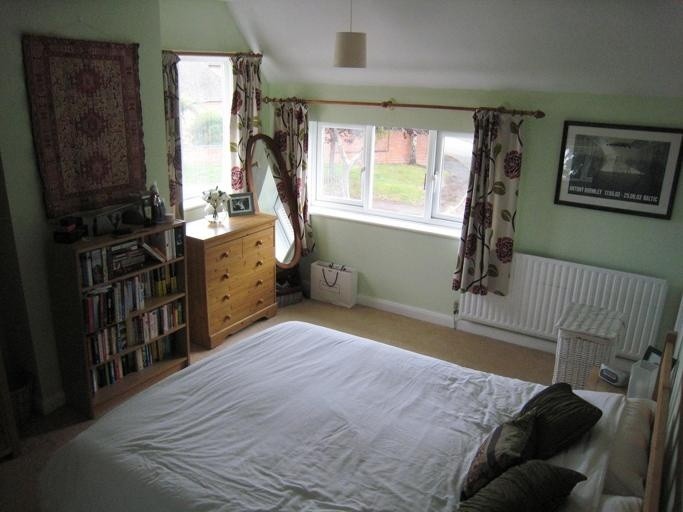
[584,365,659,401]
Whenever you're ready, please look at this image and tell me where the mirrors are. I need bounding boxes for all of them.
[245,134,301,270]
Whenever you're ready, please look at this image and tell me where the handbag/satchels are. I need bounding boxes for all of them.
[309,260,358,308]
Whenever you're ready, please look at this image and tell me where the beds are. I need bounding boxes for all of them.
[40,320,676,511]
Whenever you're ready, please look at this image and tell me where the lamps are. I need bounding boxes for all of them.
[333,1,366,68]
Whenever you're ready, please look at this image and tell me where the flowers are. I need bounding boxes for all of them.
[201,185,232,220]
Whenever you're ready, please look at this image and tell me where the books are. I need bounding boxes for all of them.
[80,226,185,397]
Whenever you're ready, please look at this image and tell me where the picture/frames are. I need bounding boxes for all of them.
[554,121,683,220]
[227,192,254,217]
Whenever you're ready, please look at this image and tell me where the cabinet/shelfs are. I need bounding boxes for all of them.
[47,220,192,420]
[185,213,279,349]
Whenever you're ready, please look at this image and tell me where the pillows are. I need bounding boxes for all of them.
[458,382,601,512]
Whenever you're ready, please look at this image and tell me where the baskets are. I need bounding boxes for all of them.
[551,302,629,391]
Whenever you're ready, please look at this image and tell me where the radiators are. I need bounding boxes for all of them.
[458,249,669,364]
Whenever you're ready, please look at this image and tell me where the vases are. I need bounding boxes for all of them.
[204,203,227,224]
